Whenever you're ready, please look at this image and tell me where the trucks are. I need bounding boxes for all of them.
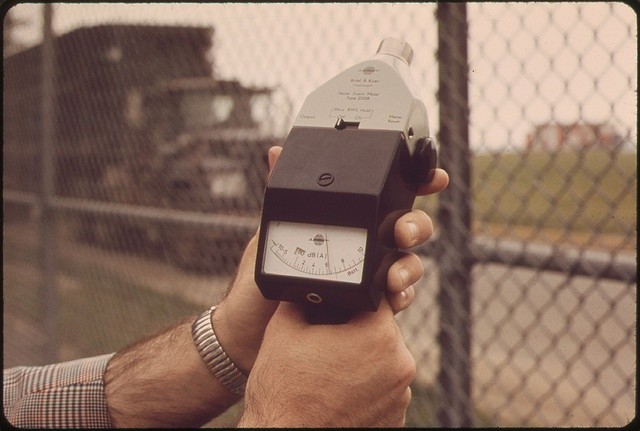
[3,25,289,270]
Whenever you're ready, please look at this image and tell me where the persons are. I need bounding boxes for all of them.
[3,146,449,428]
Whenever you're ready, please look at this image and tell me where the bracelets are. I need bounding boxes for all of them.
[192,304,247,399]
